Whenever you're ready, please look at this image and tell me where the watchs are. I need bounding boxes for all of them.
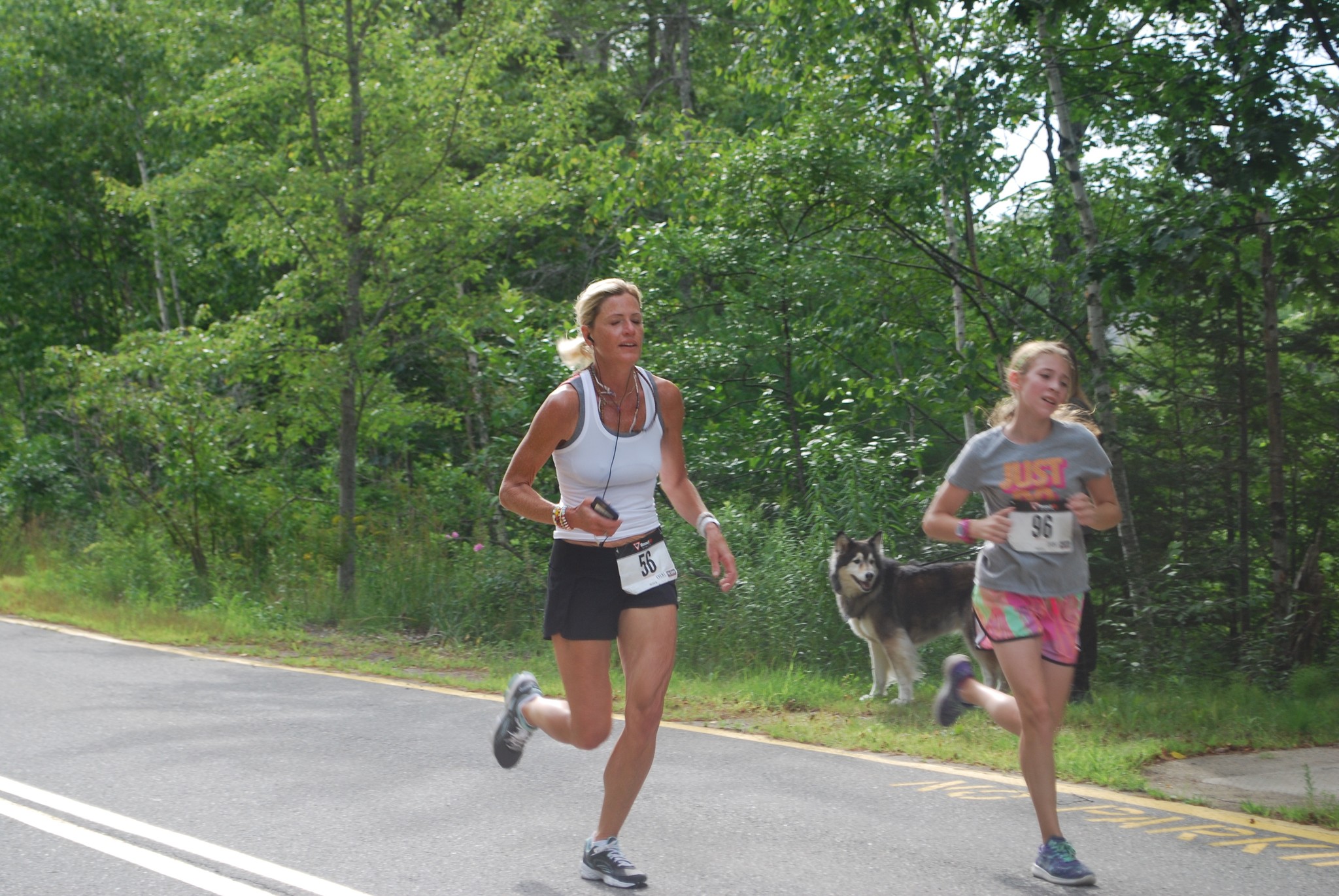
[955,519,976,543]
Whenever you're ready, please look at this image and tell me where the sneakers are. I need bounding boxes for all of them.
[490,671,543,770]
[934,654,977,728]
[1032,835,1097,886]
[579,837,648,887]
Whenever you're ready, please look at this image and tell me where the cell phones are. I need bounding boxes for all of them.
[591,496,619,520]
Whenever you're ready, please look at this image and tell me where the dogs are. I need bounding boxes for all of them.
[828,530,1098,708]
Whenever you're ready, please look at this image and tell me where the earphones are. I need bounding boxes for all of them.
[588,333,593,342]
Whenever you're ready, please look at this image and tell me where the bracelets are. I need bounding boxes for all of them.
[552,506,572,530]
[696,512,721,539]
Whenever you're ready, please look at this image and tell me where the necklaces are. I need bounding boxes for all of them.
[588,366,658,433]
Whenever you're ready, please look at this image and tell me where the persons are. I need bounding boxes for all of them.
[492,277,739,888]
[924,341,1124,886]
